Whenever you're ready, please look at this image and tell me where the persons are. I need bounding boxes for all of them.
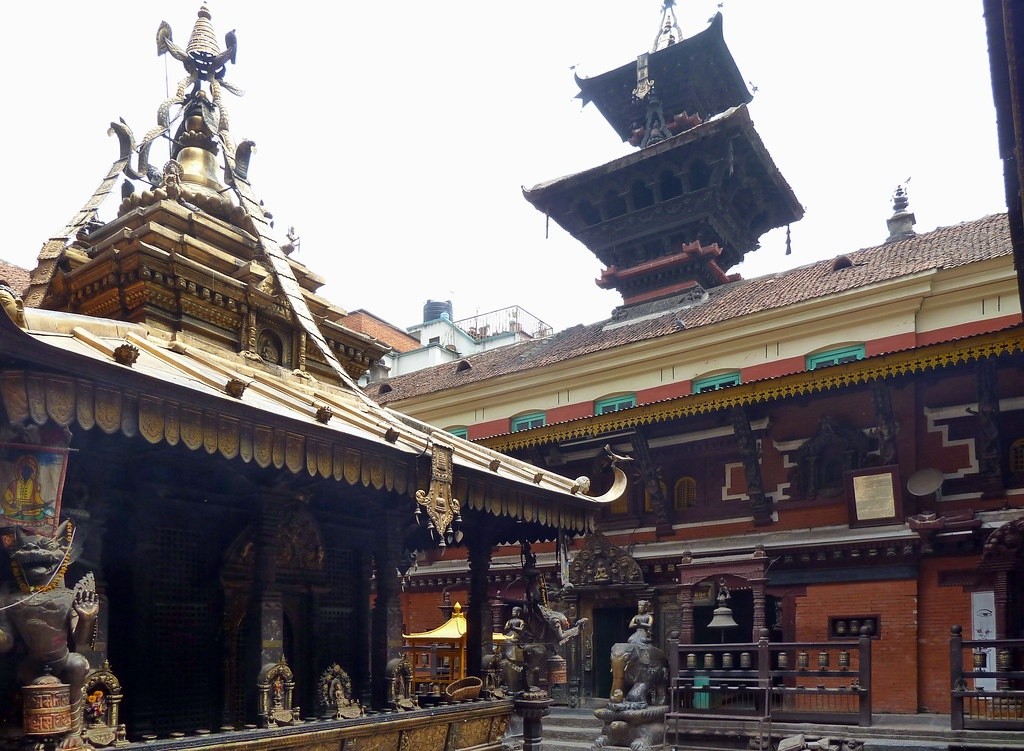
[594,562,608,579]
[627,600,654,642]
[504,607,524,643]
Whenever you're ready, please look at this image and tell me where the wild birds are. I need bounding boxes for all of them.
[121,178,135,201]
[225,29,237,64]
[147,164,163,185]
[675,318,685,329]
